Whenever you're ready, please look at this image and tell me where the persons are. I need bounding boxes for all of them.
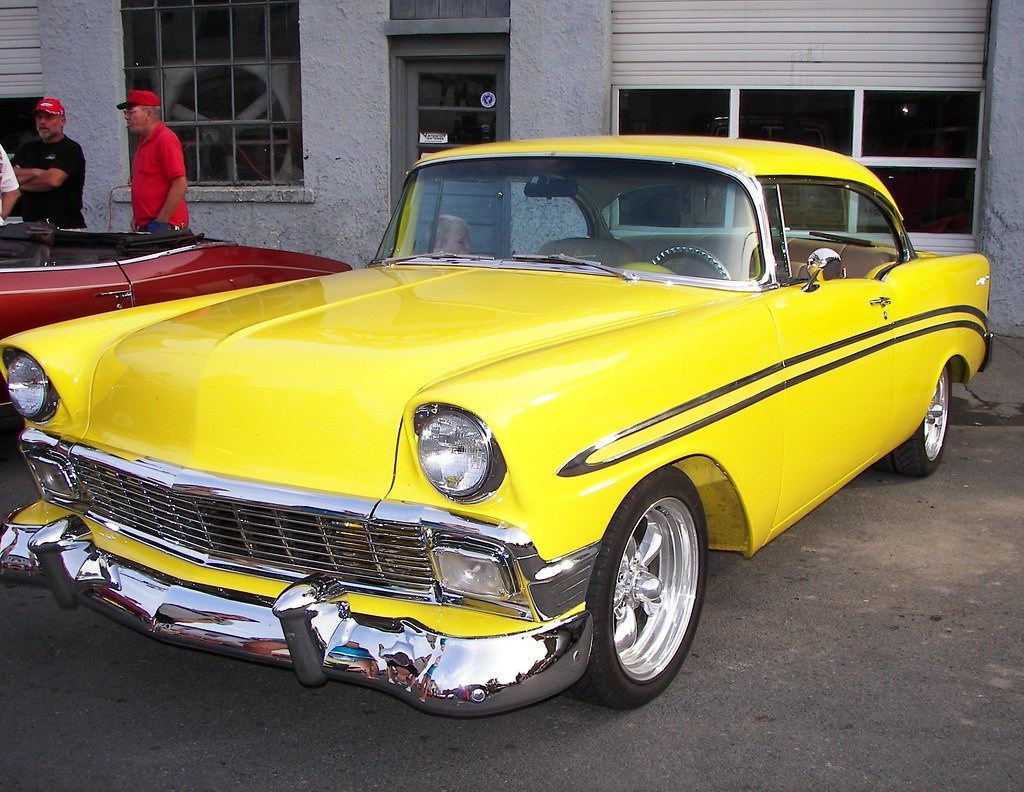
[435,215,472,256]
[9,99,87,232]
[116,90,189,234]
[808,254,825,282]
[0,145,21,228]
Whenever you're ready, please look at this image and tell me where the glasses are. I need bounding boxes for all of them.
[123,107,142,115]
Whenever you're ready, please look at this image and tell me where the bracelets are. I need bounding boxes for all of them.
[0,215,5,221]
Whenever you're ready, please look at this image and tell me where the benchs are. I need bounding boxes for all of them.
[623,235,897,283]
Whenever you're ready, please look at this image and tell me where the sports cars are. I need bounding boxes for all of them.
[0,221,357,438]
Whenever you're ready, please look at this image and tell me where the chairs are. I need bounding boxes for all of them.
[537,237,635,268]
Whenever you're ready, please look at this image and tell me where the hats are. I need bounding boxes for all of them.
[115,89,160,110]
[31,98,66,116]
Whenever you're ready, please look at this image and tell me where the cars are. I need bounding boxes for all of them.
[7,133,999,720]
